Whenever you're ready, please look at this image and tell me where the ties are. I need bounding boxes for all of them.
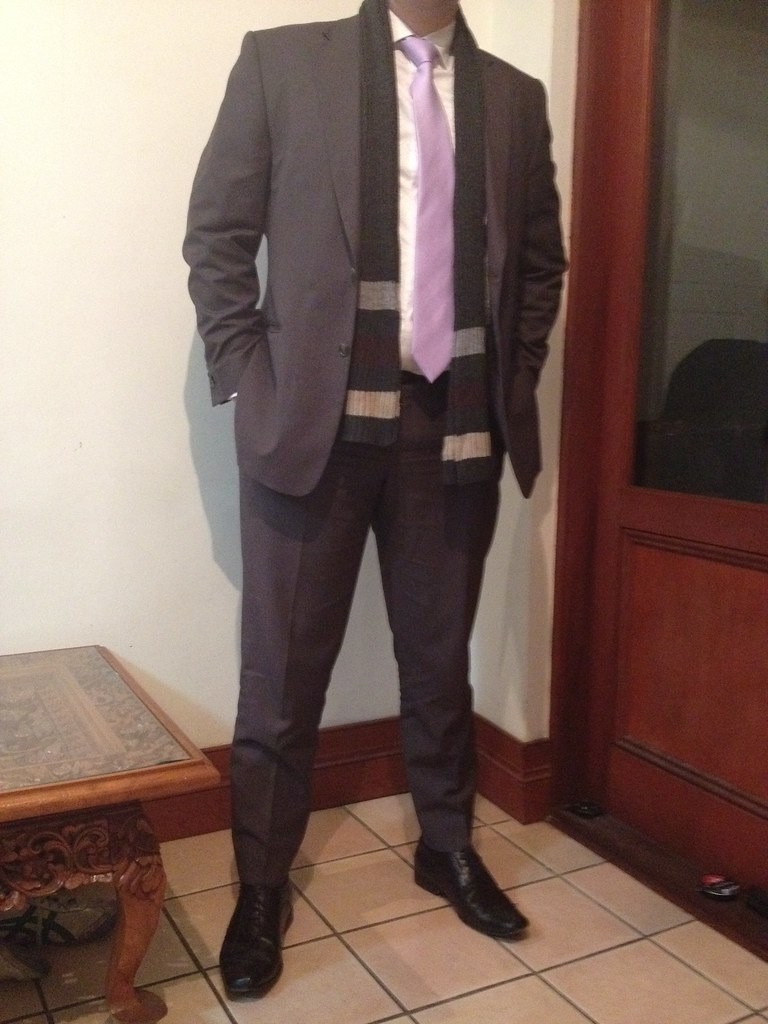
[401,38,457,382]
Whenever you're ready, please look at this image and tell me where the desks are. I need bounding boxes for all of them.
[1,644,220,1024]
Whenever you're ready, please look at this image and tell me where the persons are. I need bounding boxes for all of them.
[182,0,567,1003]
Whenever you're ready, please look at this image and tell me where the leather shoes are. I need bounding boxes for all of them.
[413,842,530,938]
[219,882,293,998]
